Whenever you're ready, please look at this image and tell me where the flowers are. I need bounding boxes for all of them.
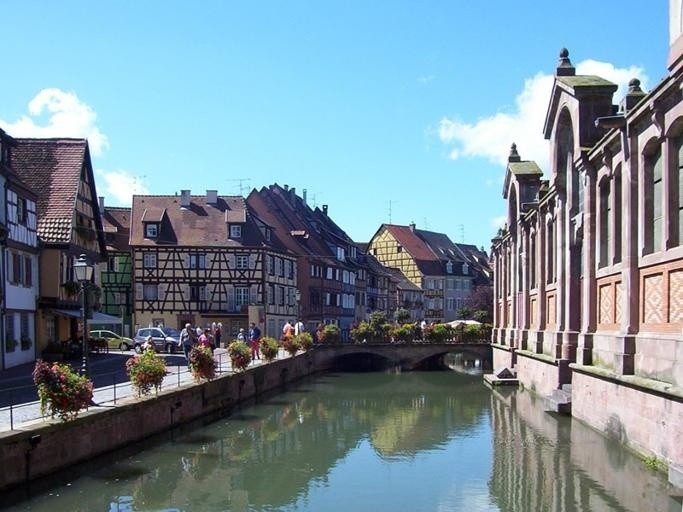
[32,359,95,422]
[123,345,170,399]
[185,330,313,385]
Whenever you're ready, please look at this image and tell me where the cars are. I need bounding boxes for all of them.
[133,327,181,354]
[87,330,134,351]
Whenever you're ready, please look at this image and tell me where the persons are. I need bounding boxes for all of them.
[178,322,198,366]
[420,320,426,337]
[198,328,215,355]
[414,319,421,340]
[214,327,221,348]
[294,319,305,336]
[248,323,262,360]
[282,321,294,336]
[237,328,248,344]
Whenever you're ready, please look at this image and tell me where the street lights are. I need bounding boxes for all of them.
[73,254,99,407]
[295,290,302,335]
